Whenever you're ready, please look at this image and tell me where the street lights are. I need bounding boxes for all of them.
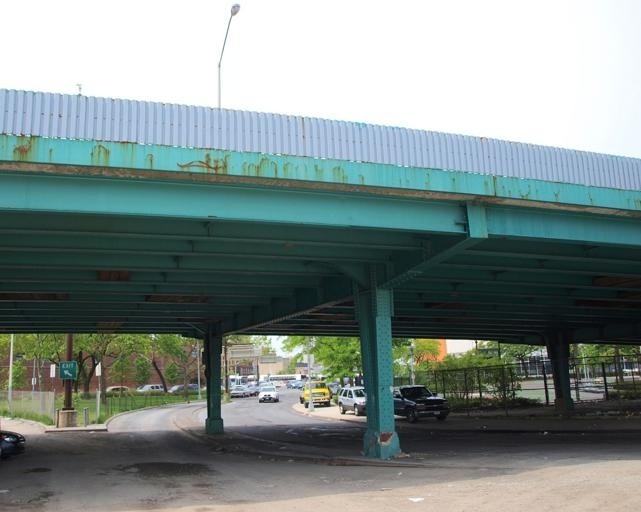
[191,337,202,401]
[218,2,241,105]
[407,342,417,388]
[255,348,259,386]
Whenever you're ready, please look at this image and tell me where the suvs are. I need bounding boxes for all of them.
[335,386,367,417]
[391,384,451,422]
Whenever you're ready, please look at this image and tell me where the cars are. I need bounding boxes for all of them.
[103,384,131,397]
[136,383,169,393]
[167,384,198,395]
[0,429,25,459]
[220,372,350,408]
